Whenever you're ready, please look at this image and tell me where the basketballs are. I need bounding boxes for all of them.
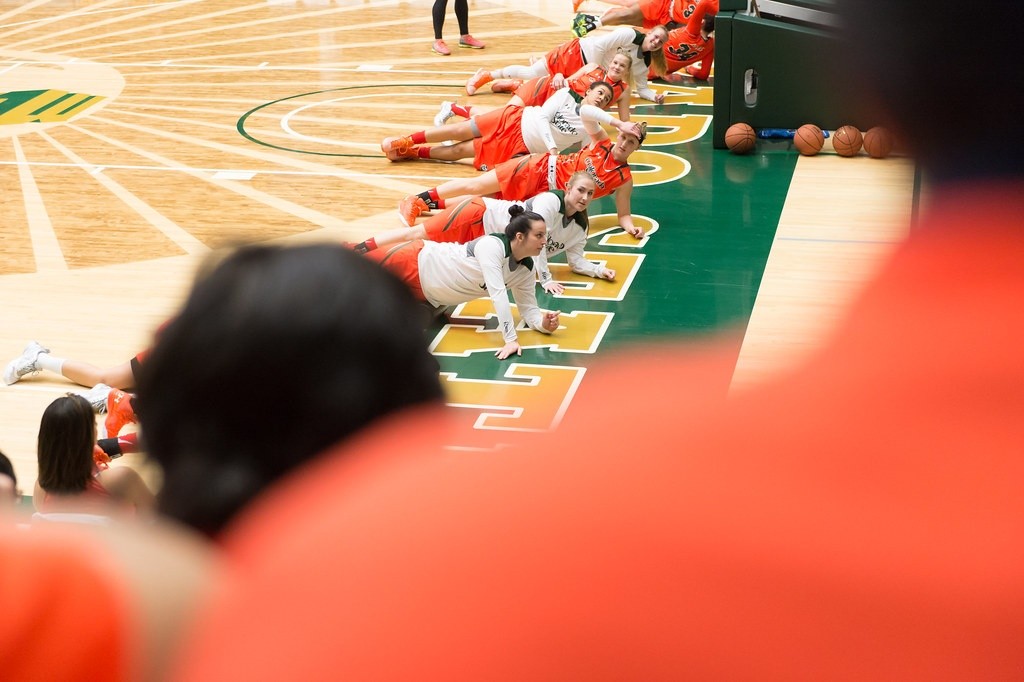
[832,126,863,157]
[864,127,893,158]
[725,123,755,152]
[794,124,824,156]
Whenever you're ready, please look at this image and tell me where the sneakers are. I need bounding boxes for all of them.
[381,133,414,152]
[491,77,523,94]
[105,387,137,439]
[571,11,587,30]
[386,145,421,160]
[4,341,50,384]
[432,38,450,56]
[466,67,495,96]
[80,382,114,415]
[572,24,588,38]
[458,33,485,49]
[434,101,456,128]
[92,444,112,468]
[398,193,430,227]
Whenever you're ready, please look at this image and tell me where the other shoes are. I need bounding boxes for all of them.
[342,241,355,253]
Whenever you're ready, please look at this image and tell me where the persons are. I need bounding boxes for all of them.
[0,1,1023,682]
